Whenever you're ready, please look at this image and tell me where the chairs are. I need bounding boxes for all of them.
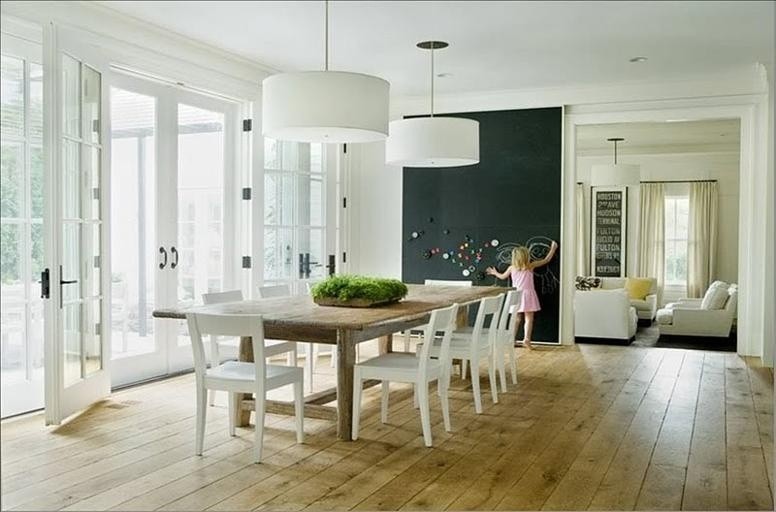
[576,288,638,346]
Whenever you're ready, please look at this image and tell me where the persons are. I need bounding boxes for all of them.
[486,241,558,350]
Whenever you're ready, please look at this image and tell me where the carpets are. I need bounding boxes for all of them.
[630,319,736,351]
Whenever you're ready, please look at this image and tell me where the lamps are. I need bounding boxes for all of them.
[590,138,640,188]
[386,42,480,169]
[262,0,389,146]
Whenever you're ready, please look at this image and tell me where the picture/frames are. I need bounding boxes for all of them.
[590,186,627,278]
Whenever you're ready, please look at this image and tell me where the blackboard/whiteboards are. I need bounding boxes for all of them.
[401,107,565,347]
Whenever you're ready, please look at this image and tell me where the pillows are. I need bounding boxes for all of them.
[576,277,602,292]
[624,277,653,300]
[702,280,730,310]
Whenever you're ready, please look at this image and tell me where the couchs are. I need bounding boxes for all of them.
[656,282,738,342]
[576,276,657,327]
